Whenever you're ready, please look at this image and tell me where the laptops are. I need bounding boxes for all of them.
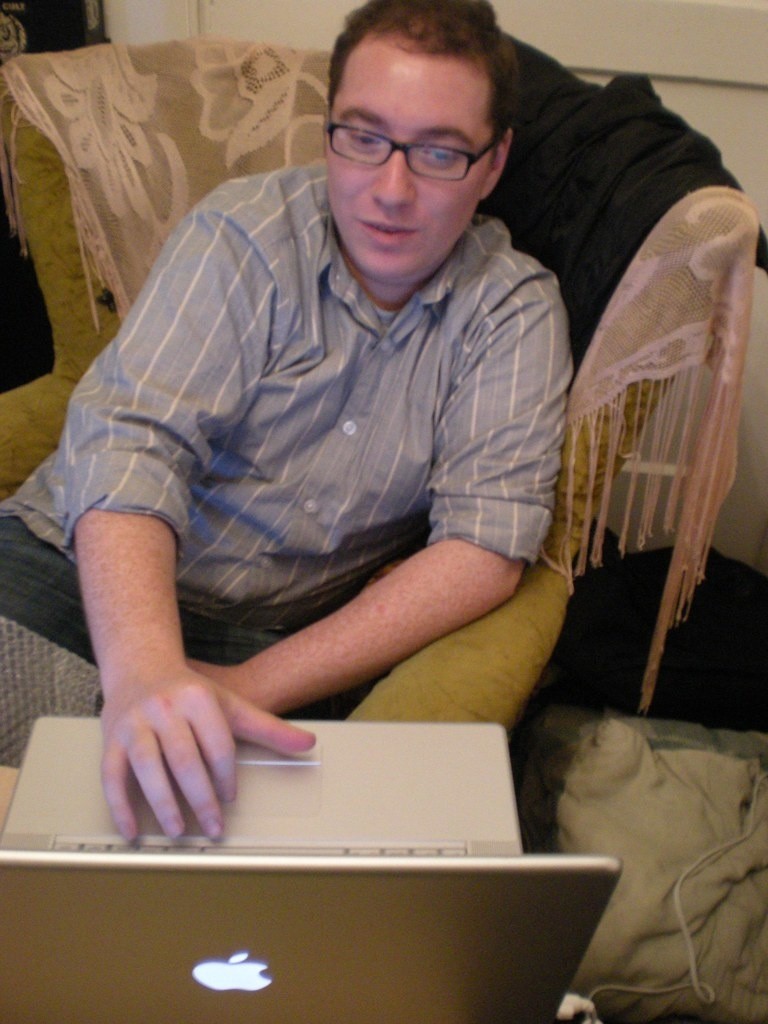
[0,714,622,1024]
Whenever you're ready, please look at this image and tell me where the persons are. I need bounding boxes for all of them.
[0,0,572,843]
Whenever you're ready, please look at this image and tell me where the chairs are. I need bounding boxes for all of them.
[0,43,767,720]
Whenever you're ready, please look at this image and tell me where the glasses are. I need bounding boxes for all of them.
[327,122,500,182]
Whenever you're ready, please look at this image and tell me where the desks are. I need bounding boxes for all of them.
[0,616,121,767]
